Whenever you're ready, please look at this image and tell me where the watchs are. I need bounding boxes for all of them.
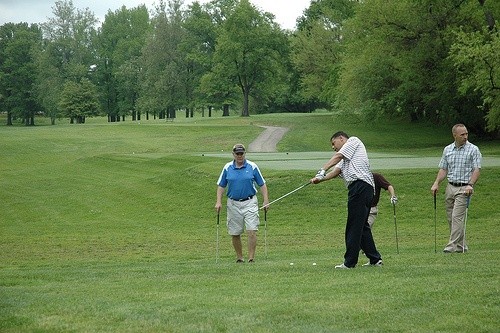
[469,184,474,187]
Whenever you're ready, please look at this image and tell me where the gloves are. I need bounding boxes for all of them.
[314,169,326,181]
[390,195,398,204]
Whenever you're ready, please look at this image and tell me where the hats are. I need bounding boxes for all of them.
[233,144,246,153]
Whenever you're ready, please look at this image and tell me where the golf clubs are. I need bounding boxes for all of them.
[434,190,437,253]
[251,182,312,219]
[393,196,399,254]
[264,208,266,260]
[215,210,220,264]
[462,195,470,254]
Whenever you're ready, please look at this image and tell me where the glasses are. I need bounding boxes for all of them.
[235,152,244,155]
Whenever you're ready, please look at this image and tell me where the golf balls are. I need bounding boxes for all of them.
[289,263,294,266]
[312,262,316,265]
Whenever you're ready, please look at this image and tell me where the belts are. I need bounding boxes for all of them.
[448,181,469,187]
[230,196,253,202]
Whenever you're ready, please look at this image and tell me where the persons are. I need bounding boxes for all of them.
[215,143,270,263]
[361,160,398,255]
[431,124,482,253]
[310,131,384,268]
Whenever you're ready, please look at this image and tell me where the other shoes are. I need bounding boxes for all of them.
[236,257,245,263]
[363,259,383,267]
[454,246,468,253]
[335,263,357,270]
[443,246,457,253]
[248,259,255,263]
